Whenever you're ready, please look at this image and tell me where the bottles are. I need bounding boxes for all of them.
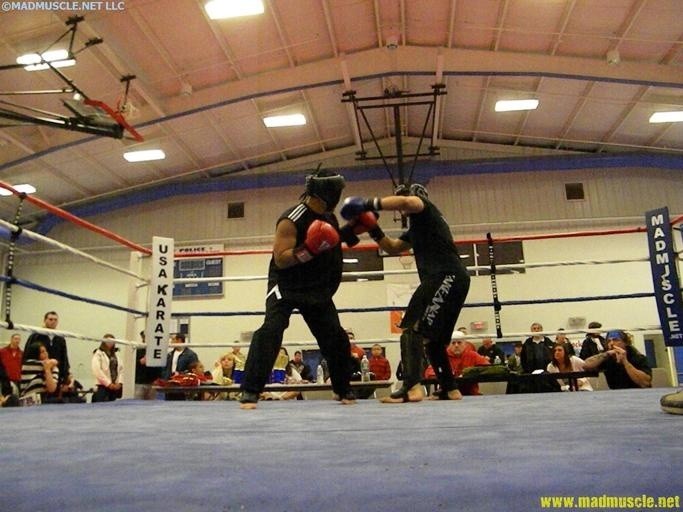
[360,354,370,381]
[494,355,501,364]
[315,364,324,383]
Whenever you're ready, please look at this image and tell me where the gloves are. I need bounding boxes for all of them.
[293,196,377,264]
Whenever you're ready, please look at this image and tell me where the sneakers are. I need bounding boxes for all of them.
[660,384,682,415]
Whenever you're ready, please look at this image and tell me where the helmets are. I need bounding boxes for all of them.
[393,183,429,200]
[305,174,345,211]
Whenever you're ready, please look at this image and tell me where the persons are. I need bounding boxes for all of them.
[270,347,333,384]
[91,334,121,402]
[0,311,87,405]
[212,352,245,383]
[397,322,653,395]
[232,340,245,362]
[239,170,379,411]
[341,182,471,402]
[346,332,366,380]
[135,331,160,399]
[368,344,391,380]
[166,333,198,400]
